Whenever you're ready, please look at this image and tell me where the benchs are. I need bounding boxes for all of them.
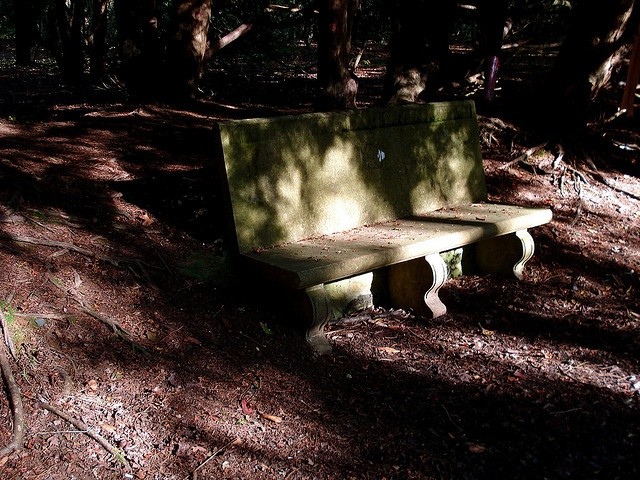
[214,99,553,360]
